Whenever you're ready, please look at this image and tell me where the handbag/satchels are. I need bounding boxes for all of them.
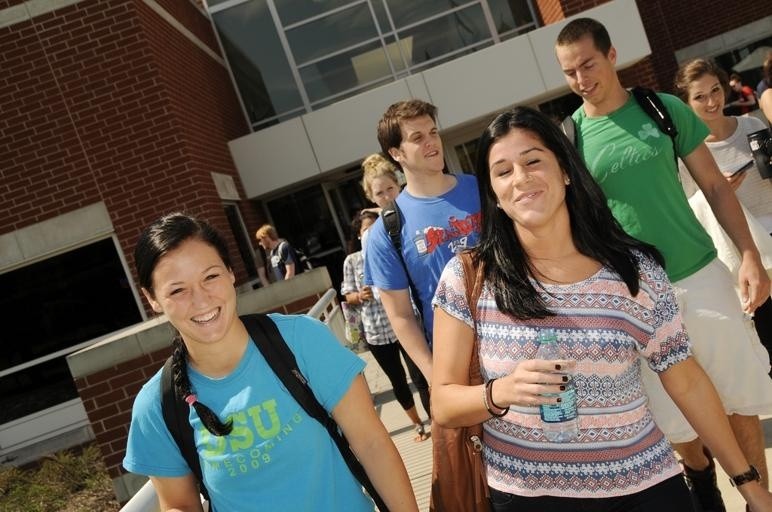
[428,377,490,511]
[342,302,366,344]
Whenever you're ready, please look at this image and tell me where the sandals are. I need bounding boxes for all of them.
[415,423,428,442]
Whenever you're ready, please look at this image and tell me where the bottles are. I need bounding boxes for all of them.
[534,327,580,444]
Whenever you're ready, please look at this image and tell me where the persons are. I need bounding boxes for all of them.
[254,224,323,287]
[123,212,419,512]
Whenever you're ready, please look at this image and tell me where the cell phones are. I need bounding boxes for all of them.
[730,158,753,177]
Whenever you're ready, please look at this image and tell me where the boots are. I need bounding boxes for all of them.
[679,447,726,511]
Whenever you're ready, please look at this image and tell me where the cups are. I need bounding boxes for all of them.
[747,127,772,180]
